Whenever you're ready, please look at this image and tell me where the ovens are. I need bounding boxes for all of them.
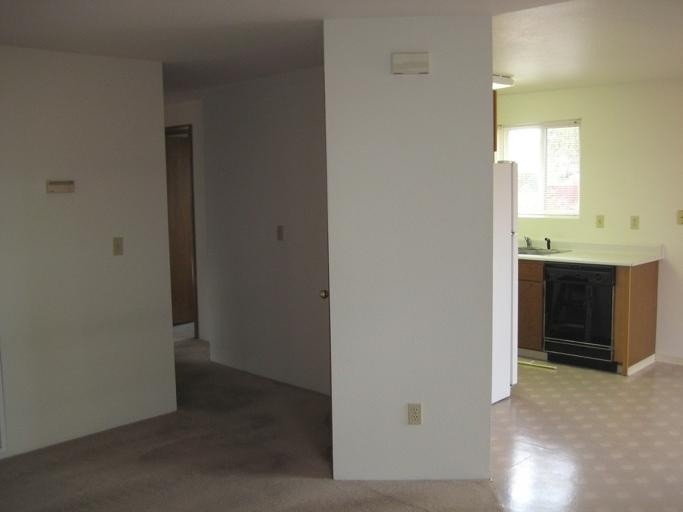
[542,264,617,373]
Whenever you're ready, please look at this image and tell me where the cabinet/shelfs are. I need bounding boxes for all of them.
[518,259,630,377]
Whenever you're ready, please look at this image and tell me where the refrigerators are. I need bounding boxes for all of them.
[491,157,519,406]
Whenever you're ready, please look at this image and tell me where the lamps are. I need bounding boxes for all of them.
[492,74,516,90]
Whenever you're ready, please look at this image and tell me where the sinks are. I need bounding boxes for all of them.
[518,248,572,255]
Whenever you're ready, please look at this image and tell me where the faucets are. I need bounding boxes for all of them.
[525,236,532,248]
[545,238,550,249]
